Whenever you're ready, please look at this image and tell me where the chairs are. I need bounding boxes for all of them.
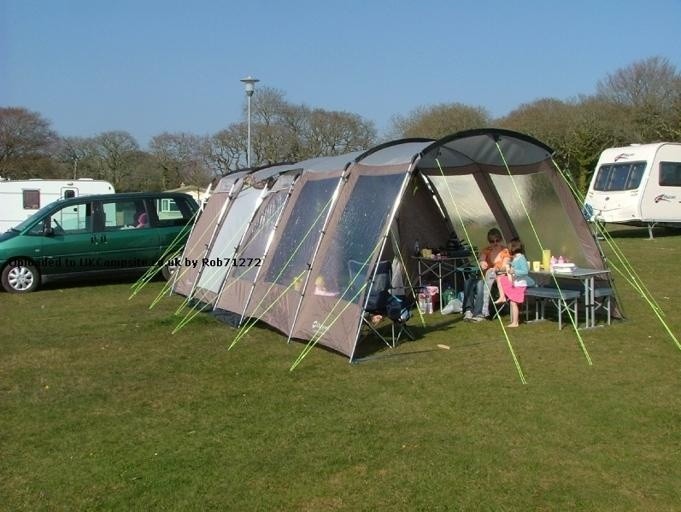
[348,258,419,348]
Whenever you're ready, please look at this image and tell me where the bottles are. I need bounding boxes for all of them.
[414,238,421,257]
[551,255,564,266]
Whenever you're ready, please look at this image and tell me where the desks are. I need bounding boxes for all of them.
[411,248,475,314]
[529,267,613,329]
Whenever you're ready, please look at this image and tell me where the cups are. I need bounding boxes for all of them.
[422,248,433,257]
[542,249,551,272]
[533,261,541,272]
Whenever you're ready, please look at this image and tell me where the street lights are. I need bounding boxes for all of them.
[239,74,262,168]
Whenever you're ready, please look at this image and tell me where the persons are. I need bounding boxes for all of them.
[493,237,530,327]
[463,228,512,322]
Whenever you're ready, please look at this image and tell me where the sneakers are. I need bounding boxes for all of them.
[462,310,473,322]
[470,314,488,322]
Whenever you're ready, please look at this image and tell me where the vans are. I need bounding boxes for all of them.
[0,191,204,293]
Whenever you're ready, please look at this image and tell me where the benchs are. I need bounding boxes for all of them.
[510,282,615,331]
